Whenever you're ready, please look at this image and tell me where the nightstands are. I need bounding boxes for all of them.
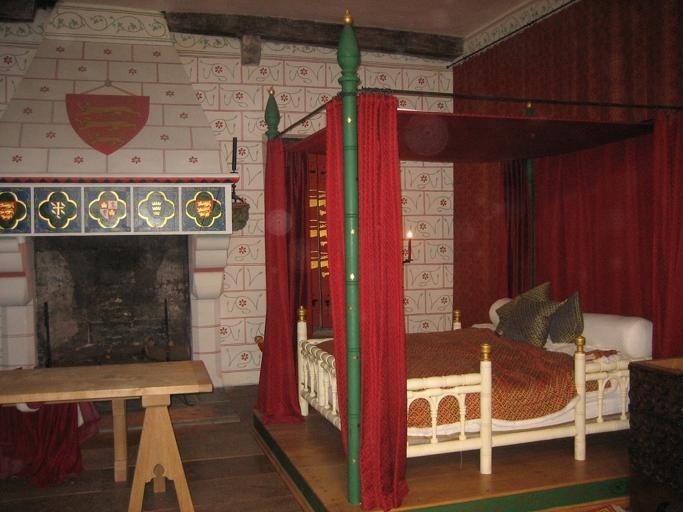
[628,358,682,512]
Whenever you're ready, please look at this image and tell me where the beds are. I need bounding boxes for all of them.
[297,306,653,474]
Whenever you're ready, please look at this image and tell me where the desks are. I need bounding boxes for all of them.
[0,360,212,512]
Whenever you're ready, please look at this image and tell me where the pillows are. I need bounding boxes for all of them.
[489,281,653,361]
